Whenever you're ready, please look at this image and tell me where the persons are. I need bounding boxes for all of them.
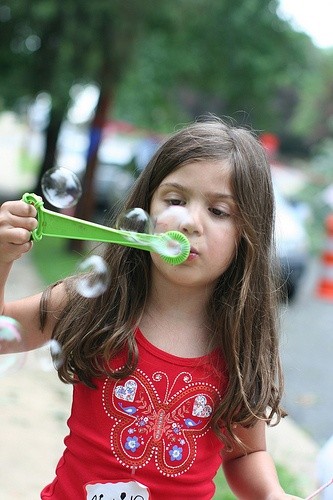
[0,112,314,500]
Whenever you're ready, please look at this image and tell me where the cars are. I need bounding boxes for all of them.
[110,152,313,307]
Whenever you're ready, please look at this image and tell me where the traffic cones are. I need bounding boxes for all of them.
[308,214,332,301]
